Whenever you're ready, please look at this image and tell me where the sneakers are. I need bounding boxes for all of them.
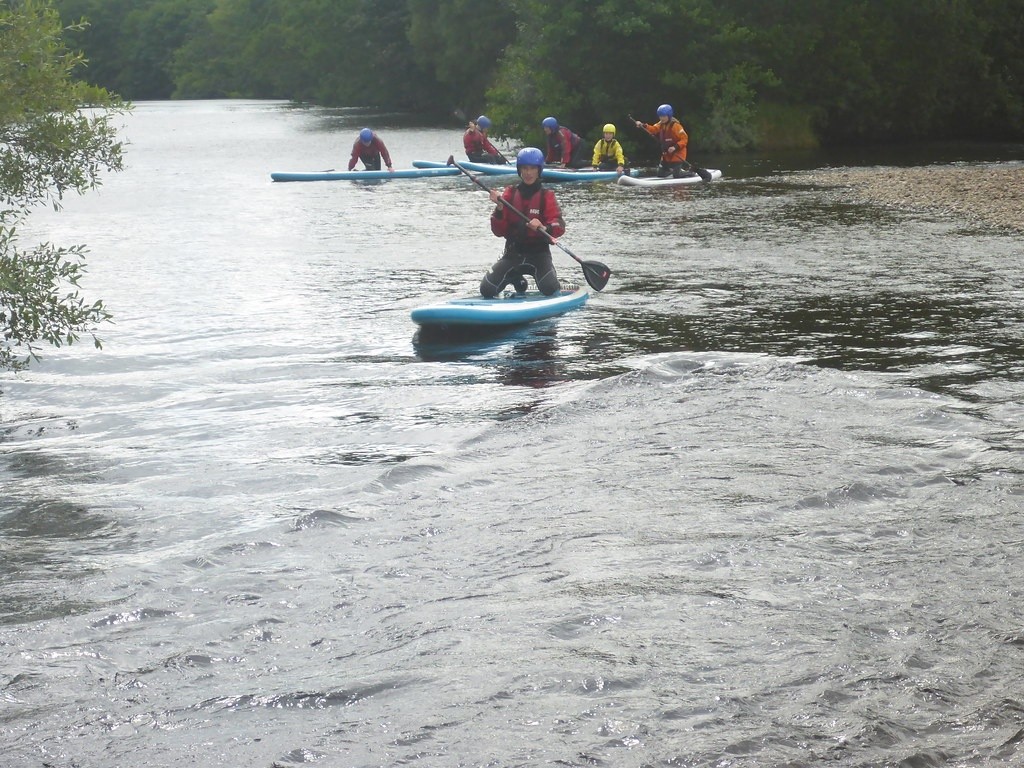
[514,275,527,292]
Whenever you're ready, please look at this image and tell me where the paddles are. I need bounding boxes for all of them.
[475,128,513,165]
[445,154,611,292]
[626,113,713,182]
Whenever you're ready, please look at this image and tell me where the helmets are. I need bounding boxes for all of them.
[603,124,616,137]
[657,104,673,119]
[517,147,544,176]
[360,128,372,141]
[477,116,490,130]
[542,117,557,129]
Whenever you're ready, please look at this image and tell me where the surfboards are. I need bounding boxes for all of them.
[270,167,462,181]
[541,167,639,181]
[617,169,722,187]
[411,278,589,325]
[456,160,600,175]
[412,159,518,169]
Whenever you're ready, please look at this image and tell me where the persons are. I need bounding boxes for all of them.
[542,117,592,169]
[348,128,394,172]
[636,104,695,178]
[464,115,506,165]
[592,124,631,178]
[479,147,565,298]
